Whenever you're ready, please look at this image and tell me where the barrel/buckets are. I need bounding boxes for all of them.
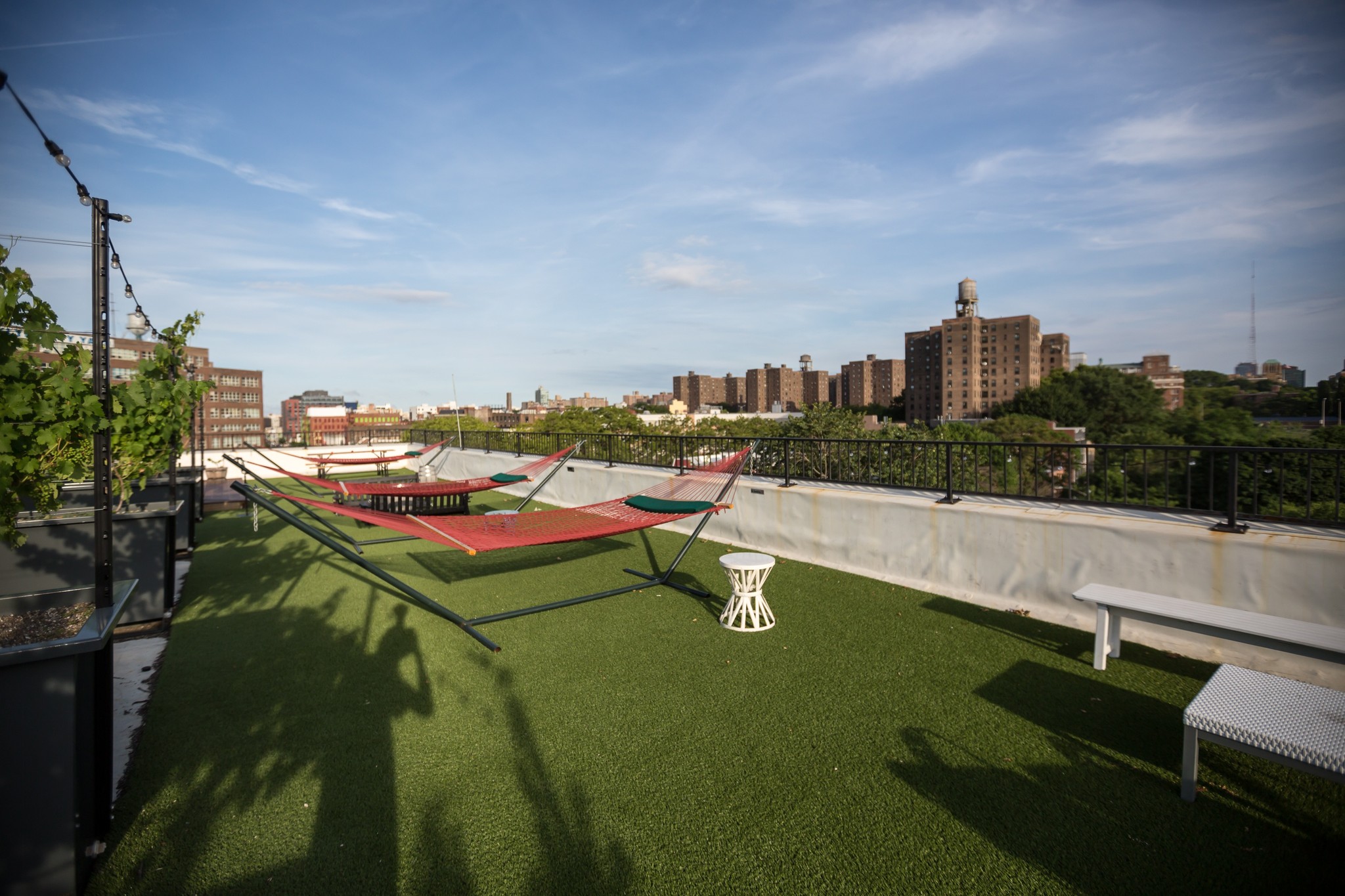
[419,465,437,483]
[205,467,227,480]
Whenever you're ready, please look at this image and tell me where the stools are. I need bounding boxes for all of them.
[719,552,776,633]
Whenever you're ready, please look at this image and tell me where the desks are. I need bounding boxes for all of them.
[1180,663,1345,804]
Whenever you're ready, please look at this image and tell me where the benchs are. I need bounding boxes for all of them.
[333,476,471,523]
[1072,583,1345,670]
